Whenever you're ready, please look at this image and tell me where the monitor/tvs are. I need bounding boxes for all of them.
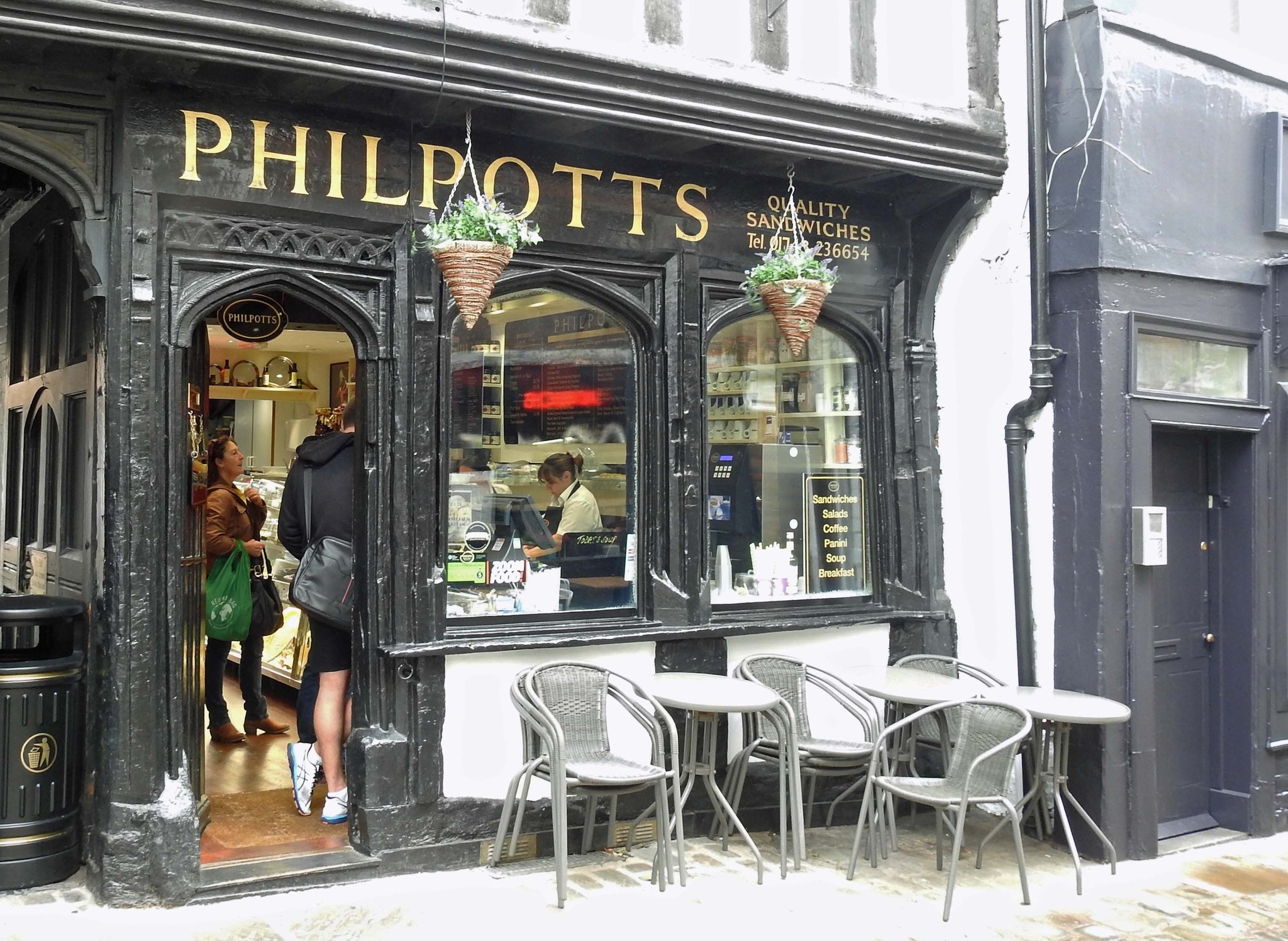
[484,493,556,550]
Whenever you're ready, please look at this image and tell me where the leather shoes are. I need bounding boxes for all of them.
[207,721,245,742]
[244,717,290,735]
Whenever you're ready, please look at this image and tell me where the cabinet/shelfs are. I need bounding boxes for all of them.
[482,353,503,449]
[705,358,863,470]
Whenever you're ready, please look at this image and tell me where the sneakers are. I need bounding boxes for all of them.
[287,743,321,816]
[321,786,349,824]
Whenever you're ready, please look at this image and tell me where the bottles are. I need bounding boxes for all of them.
[846,434,862,463]
[781,372,799,413]
[223,359,230,386]
[257,366,270,387]
[785,532,799,595]
[291,363,302,389]
[706,336,794,368]
[797,371,817,412]
[210,375,216,385]
[833,435,848,463]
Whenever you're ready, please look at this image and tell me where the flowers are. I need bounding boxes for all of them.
[739,240,840,314]
[408,194,543,254]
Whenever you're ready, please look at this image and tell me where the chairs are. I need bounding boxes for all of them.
[492,659,689,907]
[711,653,1051,917]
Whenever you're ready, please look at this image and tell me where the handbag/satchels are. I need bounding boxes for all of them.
[250,548,284,635]
[205,540,253,640]
[288,536,352,631]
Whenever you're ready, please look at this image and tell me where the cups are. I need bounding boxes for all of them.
[844,382,858,411]
[831,382,846,411]
[733,572,758,597]
[714,545,732,597]
[772,578,787,597]
[755,578,771,596]
[815,393,833,412]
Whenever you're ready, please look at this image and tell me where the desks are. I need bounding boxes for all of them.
[975,686,1131,896]
[625,672,780,886]
[825,664,980,871]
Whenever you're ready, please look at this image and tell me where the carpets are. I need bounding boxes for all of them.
[200,784,349,864]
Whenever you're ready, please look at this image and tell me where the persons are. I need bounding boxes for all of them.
[277,398,357,824]
[522,452,603,561]
[204,437,291,743]
[458,455,513,495]
